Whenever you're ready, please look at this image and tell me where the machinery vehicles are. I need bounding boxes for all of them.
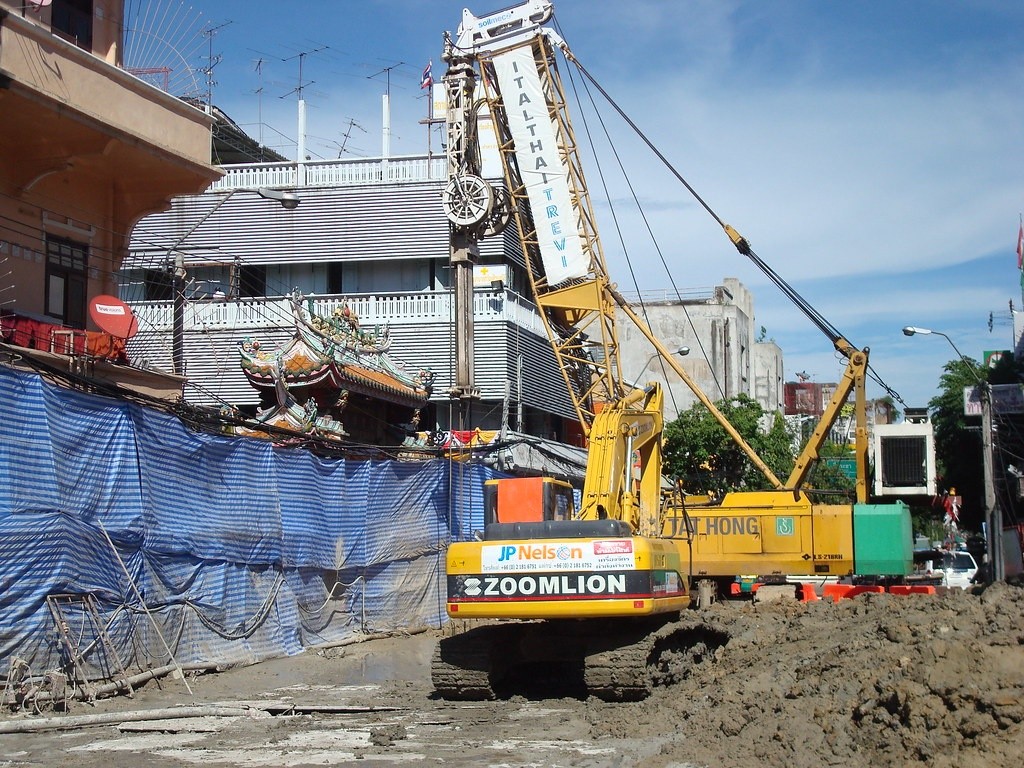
[417,0,739,703]
[547,17,955,600]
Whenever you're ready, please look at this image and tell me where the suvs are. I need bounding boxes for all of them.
[912,550,980,590]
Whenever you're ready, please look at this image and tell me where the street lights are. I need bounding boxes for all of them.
[163,188,303,378]
[901,325,1000,583]
[624,345,692,399]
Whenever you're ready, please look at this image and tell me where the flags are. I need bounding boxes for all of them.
[420,61,433,89]
